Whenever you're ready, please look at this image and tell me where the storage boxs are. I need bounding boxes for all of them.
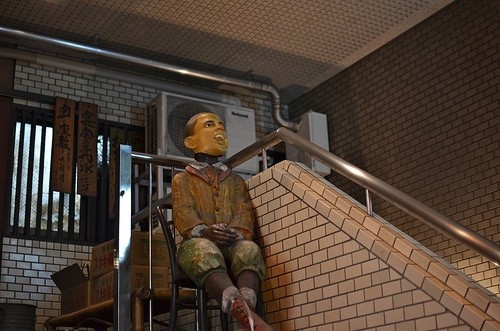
[49,231,174,316]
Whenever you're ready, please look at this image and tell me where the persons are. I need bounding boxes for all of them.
[175,110,276,330]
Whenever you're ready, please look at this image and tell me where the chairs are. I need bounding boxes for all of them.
[155,204,230,331]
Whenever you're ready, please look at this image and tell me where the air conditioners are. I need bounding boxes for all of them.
[143,92,259,184]
